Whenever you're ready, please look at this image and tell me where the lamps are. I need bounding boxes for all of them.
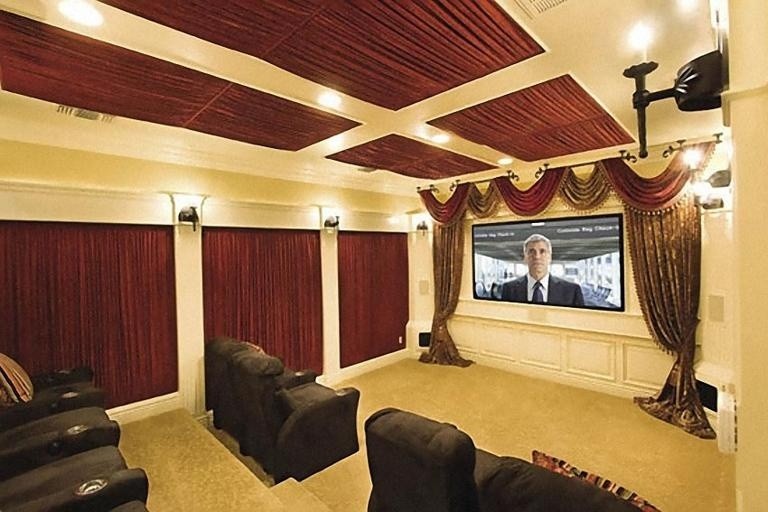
[416,220,428,236]
[624,8,730,209]
[324,216,340,231]
[179,207,199,232]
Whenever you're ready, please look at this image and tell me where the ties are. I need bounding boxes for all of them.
[532,283,543,301]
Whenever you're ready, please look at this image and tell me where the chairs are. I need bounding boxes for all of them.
[206,336,359,484]
[365,408,645,511]
[0,367,148,512]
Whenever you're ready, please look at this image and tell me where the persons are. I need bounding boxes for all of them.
[501,234,585,306]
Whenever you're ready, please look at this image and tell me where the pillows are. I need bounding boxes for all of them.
[0,353,34,404]
[533,450,661,512]
